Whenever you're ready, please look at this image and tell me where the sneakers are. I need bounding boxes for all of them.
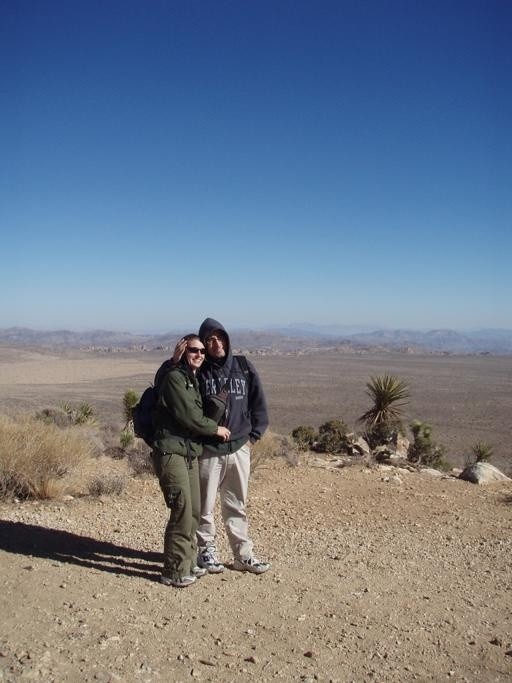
[191,565,209,577]
[233,555,271,574]
[160,574,198,587]
[197,552,225,573]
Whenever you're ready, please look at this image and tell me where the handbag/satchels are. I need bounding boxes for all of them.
[205,390,228,425]
[132,367,190,443]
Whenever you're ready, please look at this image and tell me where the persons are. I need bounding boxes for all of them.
[151,333,230,588]
[154,318,270,575]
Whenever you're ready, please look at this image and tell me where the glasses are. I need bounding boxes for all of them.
[186,346,206,354]
